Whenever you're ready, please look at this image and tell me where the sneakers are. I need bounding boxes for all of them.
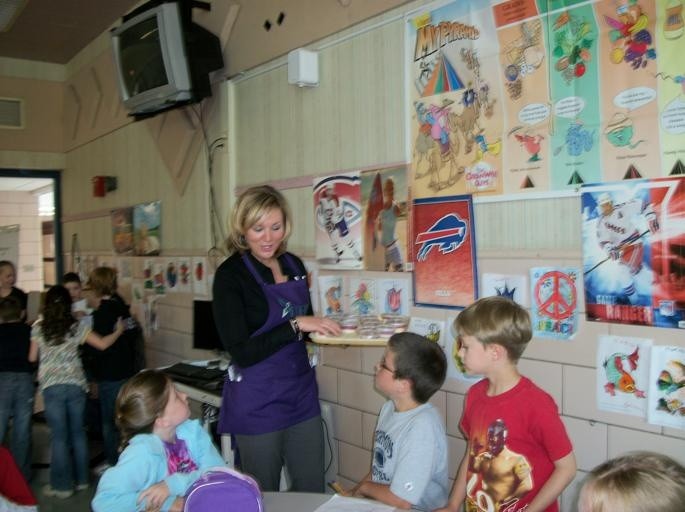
[42,464,110,498]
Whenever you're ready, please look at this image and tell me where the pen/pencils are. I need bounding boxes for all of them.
[327,482,337,492]
[331,480,344,493]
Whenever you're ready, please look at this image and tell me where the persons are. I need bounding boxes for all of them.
[594,192,662,314]
[467,416,534,511]
[431,297,577,512]
[209,181,347,494]
[0,257,150,501]
[573,451,685,511]
[374,174,404,273]
[318,181,362,262]
[338,332,448,512]
[89,368,237,511]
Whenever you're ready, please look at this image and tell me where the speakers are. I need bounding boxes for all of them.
[287,48,319,88]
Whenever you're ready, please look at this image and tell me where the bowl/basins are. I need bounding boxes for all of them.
[324,312,409,339]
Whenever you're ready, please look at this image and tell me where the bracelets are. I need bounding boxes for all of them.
[289,315,303,341]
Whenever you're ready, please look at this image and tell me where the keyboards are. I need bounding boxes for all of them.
[166,361,224,386]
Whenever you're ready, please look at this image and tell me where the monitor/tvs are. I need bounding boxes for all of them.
[110,0,222,115]
[194,301,228,365]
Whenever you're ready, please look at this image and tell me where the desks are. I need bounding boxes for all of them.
[139,361,236,471]
[261,491,407,512]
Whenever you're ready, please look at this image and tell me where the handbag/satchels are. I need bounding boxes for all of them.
[184,467,263,512]
